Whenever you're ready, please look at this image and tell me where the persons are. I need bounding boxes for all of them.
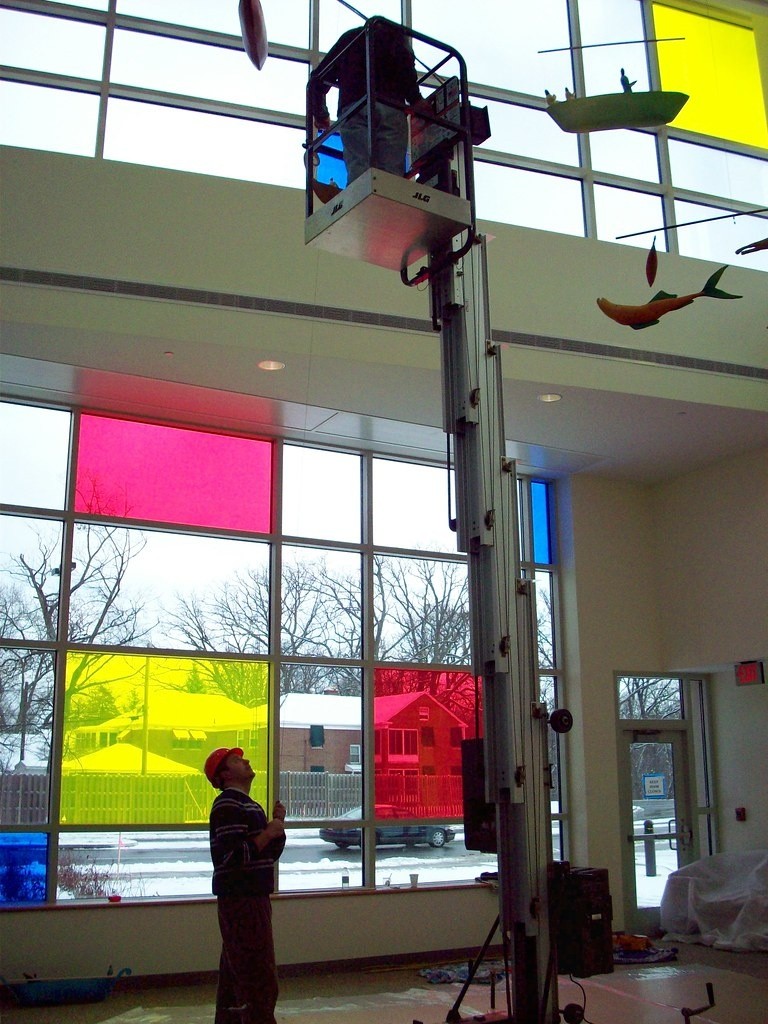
[305,15,435,190]
[204,744,288,1024]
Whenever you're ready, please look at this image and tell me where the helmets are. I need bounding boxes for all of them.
[204,748,244,788]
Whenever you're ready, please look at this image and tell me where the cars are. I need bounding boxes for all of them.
[319,804,455,850]
